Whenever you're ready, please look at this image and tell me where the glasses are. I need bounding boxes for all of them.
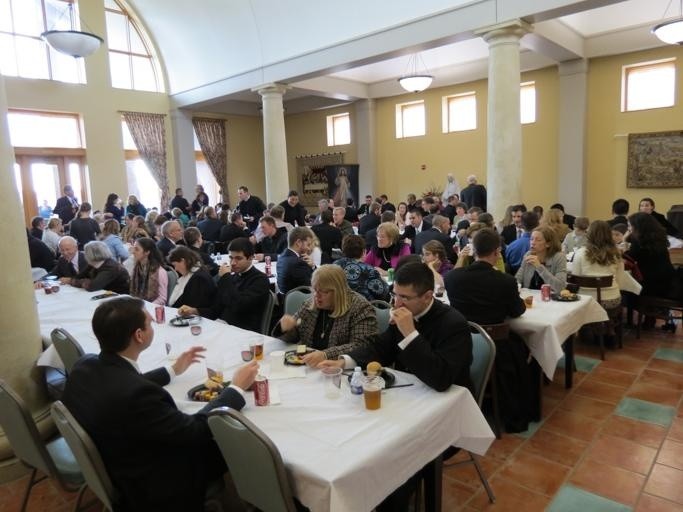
[389,290,426,301]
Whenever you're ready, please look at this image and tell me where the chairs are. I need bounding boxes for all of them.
[282,285,316,321]
[369,299,394,339]
[564,266,620,360]
[207,407,292,511]
[47,398,124,512]
[0,380,60,512]
[261,289,277,334]
[164,263,176,303]
[398,322,498,511]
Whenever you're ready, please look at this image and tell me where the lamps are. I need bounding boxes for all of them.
[42,3,107,63]
[397,55,434,95]
[648,0,683,46]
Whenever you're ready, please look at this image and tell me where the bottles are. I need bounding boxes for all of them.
[265,265,271,274]
[265,256,271,266]
[156,307,165,325]
[388,268,395,283]
[254,376,269,406]
[211,253,215,262]
[217,253,222,266]
[350,366,364,410]
[541,284,551,302]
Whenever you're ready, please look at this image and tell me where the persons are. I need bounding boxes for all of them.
[30,217,45,237]
[34,236,90,289]
[179,238,270,333]
[70,202,103,251]
[317,263,473,466]
[310,197,355,243]
[551,203,576,231]
[131,237,168,307]
[155,220,183,259]
[171,187,192,218]
[572,219,625,350]
[332,234,393,310]
[27,226,55,271]
[500,205,528,248]
[251,217,288,263]
[363,221,412,275]
[53,185,79,227]
[281,191,306,227]
[444,227,527,330]
[64,295,261,512]
[603,198,630,235]
[639,198,680,238]
[166,246,216,320]
[236,187,267,226]
[516,225,568,295]
[335,169,353,207]
[254,195,306,245]
[120,214,136,241]
[460,174,487,212]
[421,239,453,288]
[453,223,505,272]
[104,193,124,225]
[504,212,541,273]
[98,218,129,263]
[184,226,220,278]
[612,224,629,244]
[192,193,207,216]
[540,208,572,244]
[621,212,678,334]
[562,217,589,256]
[534,206,543,222]
[61,241,130,296]
[144,194,249,246]
[272,262,378,366]
[196,186,210,206]
[130,216,150,244]
[42,218,63,254]
[358,195,495,245]
[276,227,319,294]
[38,199,54,219]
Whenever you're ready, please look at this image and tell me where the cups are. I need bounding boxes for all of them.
[189,318,202,336]
[43,282,52,294]
[434,283,444,298]
[320,367,343,398]
[249,335,264,359]
[361,376,385,410]
[523,293,533,308]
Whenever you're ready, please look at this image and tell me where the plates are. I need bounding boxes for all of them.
[170,316,195,327]
[284,350,317,365]
[347,371,396,389]
[188,382,231,402]
[551,293,581,302]
